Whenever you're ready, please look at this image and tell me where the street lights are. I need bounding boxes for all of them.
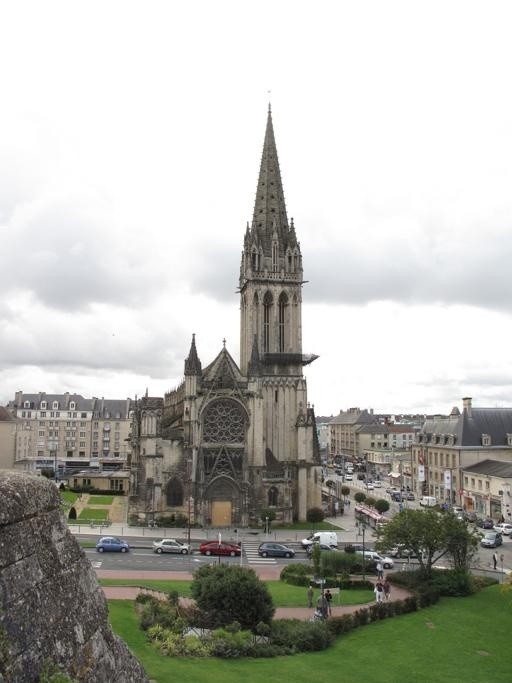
[355,515,368,580]
[188,478,192,544]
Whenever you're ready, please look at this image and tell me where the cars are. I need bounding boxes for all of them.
[96,536,129,553]
[386,486,414,502]
[453,507,512,548]
[153,538,241,556]
[351,544,425,569]
[258,542,295,558]
[307,543,338,556]
[327,461,381,490]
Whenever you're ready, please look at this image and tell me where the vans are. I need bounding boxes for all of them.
[301,532,338,549]
[420,496,436,508]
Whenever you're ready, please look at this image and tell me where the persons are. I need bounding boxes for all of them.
[316,593,328,619]
[376,561,384,580]
[493,551,498,569]
[324,590,332,607]
[383,580,391,601]
[374,583,384,602]
[307,586,313,608]
